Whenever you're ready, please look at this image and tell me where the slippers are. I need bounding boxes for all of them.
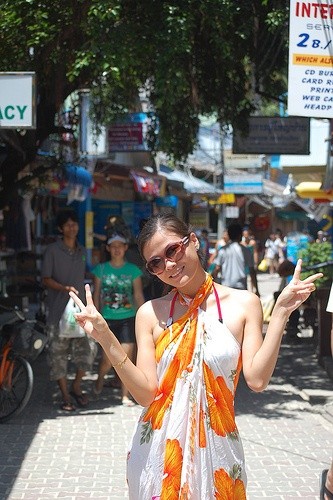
[70,390,89,407]
[60,401,75,410]
[319,469,333,500]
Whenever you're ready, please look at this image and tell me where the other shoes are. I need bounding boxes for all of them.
[122,400,136,407]
[92,387,102,400]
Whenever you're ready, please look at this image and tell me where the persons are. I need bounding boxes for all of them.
[87,230,146,407]
[40,213,98,410]
[68,213,323,500]
[195,228,330,298]
[320,278,333,499]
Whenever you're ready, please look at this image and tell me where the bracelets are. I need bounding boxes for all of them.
[112,353,127,368]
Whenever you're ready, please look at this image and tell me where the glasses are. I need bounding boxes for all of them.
[145,236,190,275]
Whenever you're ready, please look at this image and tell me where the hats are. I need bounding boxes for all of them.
[107,231,127,244]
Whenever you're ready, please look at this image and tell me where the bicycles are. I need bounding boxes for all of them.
[0,304,49,424]
